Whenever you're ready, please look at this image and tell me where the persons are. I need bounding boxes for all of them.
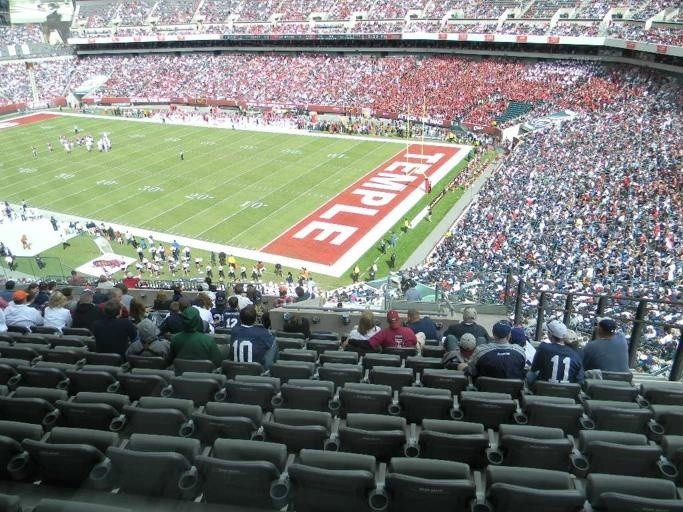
[1,200,682,389]
[0,0,682,160]
[445,161,682,200]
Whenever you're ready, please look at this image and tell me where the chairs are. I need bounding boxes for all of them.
[0,276,682,512]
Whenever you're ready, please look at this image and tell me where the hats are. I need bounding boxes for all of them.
[387,310,401,328]
[460,307,478,352]
[215,292,226,305]
[547,315,618,345]
[12,290,30,301]
[493,319,526,345]
